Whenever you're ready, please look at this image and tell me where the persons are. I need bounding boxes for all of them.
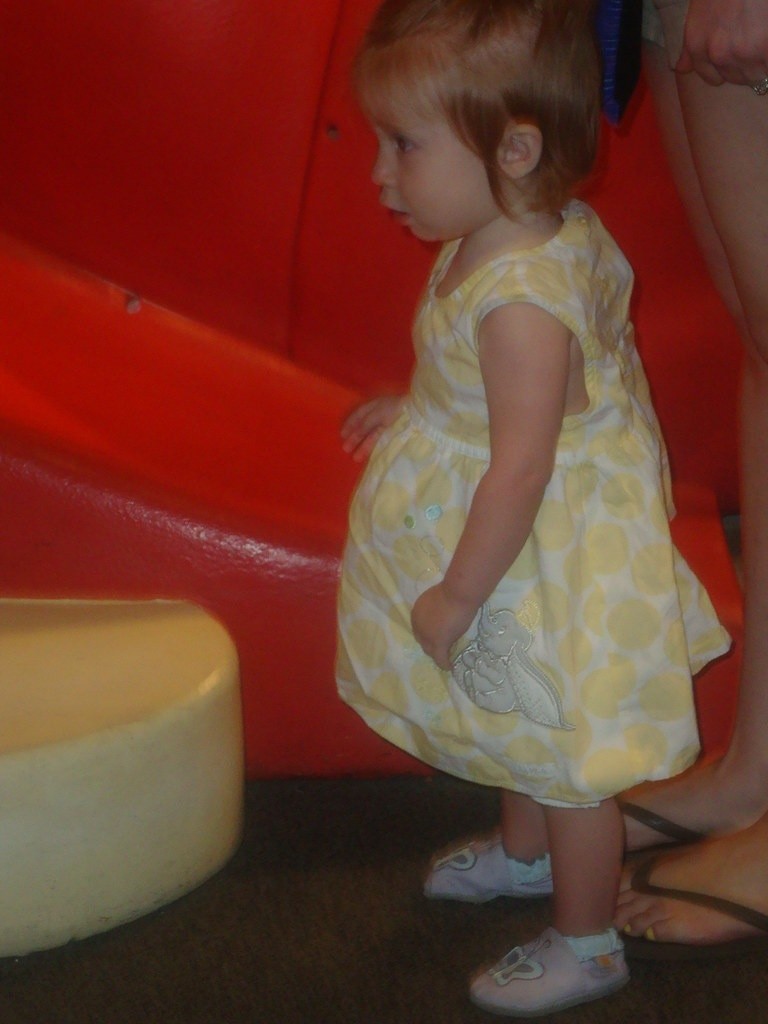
[613,1,768,948]
[335,0,737,1018]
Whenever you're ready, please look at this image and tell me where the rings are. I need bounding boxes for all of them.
[748,79,768,98]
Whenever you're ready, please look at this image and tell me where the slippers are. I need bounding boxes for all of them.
[612,862,768,961]
[616,801,711,852]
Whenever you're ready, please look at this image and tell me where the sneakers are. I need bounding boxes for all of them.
[467,928,630,1018]
[423,831,552,904]
